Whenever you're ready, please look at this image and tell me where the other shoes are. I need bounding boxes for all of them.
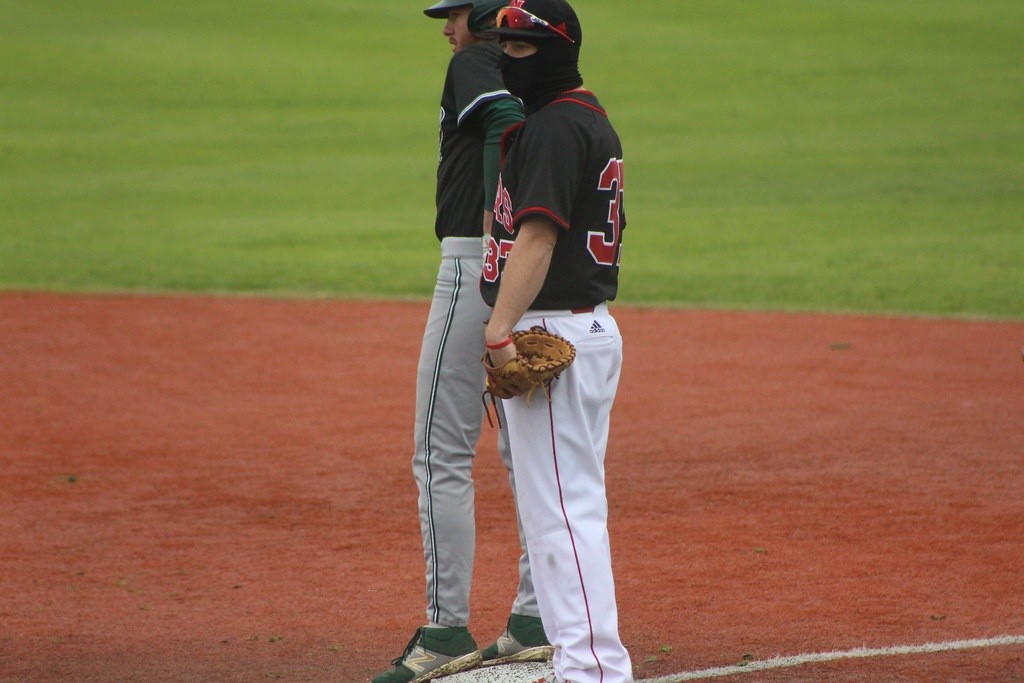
[530,672,568,683]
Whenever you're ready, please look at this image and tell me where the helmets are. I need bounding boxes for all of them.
[422,0,512,40]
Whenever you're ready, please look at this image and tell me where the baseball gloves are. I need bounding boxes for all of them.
[481,328,577,399]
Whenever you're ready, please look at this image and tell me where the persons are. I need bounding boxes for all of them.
[371,0,556,683]
[476,0,633,682]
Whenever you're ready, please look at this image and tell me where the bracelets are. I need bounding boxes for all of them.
[484,337,512,349]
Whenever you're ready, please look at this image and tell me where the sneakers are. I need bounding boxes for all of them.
[480,613,553,665]
[371,625,482,683]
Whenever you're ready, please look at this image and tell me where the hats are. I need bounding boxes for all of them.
[484,0,582,46]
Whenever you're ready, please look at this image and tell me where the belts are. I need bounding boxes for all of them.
[571,306,595,315]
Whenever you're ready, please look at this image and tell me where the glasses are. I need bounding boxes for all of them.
[496,6,577,45]
